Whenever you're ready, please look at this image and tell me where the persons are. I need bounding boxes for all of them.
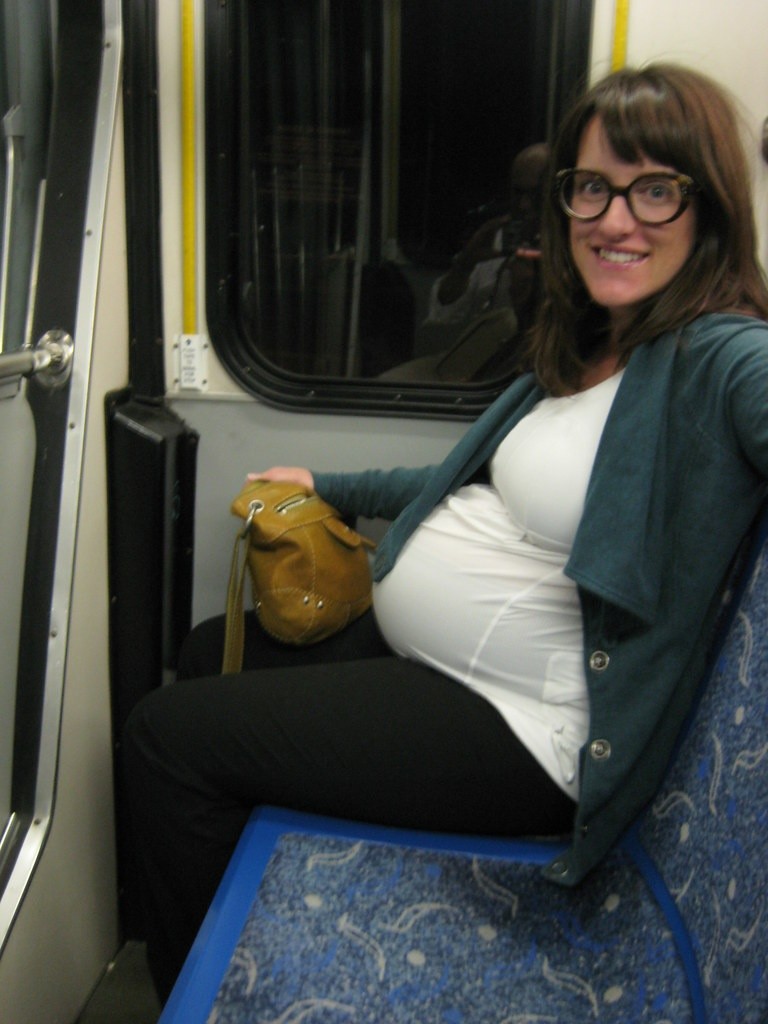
[125,61,768,1010]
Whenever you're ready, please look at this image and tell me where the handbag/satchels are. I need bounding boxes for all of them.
[222,480,377,674]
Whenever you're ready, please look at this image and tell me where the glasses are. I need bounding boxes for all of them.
[555,168,696,226]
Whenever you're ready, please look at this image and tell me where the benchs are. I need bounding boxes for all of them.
[160,531,767,1024]
